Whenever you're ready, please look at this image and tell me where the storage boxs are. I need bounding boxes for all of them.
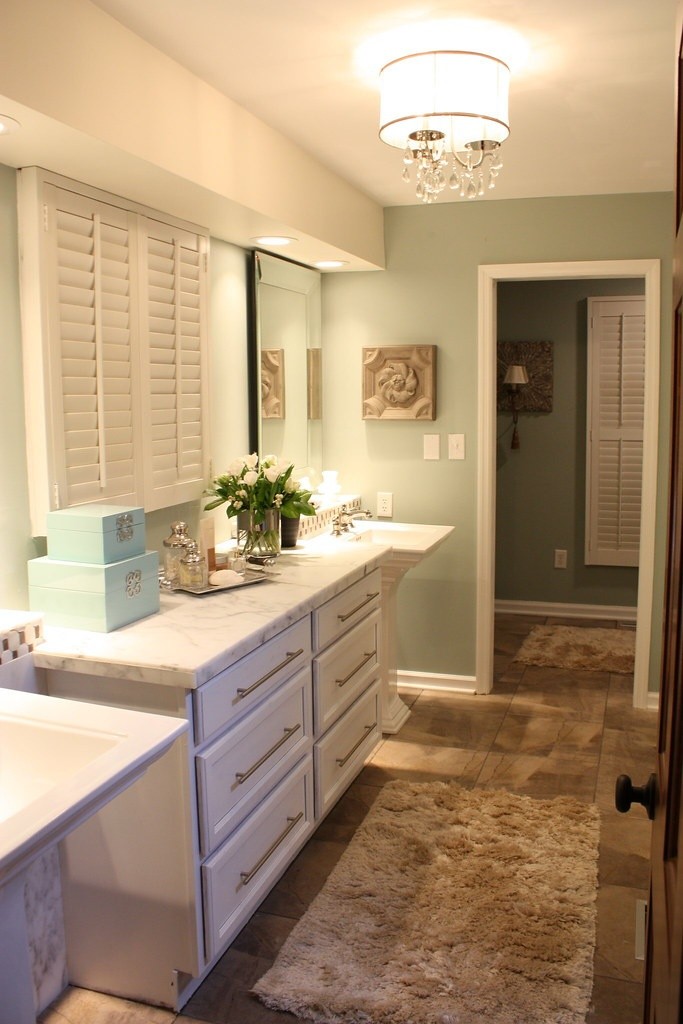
[28,505,159,633]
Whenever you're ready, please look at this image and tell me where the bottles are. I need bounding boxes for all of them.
[162,522,197,582]
[177,540,210,590]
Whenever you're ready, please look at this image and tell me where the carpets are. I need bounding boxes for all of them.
[512,623,636,675]
[251,780,598,1024]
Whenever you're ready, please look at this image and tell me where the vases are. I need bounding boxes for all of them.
[238,511,282,558]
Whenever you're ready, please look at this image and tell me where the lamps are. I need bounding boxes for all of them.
[503,365,529,450]
[378,46,511,201]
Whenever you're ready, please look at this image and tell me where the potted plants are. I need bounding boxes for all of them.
[281,482,316,548]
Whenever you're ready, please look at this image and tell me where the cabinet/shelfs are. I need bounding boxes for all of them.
[191,570,381,972]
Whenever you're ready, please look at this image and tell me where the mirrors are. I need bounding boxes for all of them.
[254,250,321,492]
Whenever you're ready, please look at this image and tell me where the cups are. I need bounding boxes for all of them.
[229,550,245,575]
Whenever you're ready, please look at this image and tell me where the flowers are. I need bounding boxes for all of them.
[218,455,304,506]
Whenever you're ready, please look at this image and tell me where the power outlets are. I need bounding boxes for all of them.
[377,492,392,517]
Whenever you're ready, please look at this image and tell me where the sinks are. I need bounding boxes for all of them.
[347,524,455,555]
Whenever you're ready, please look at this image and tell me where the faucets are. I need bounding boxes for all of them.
[330,504,372,537]
